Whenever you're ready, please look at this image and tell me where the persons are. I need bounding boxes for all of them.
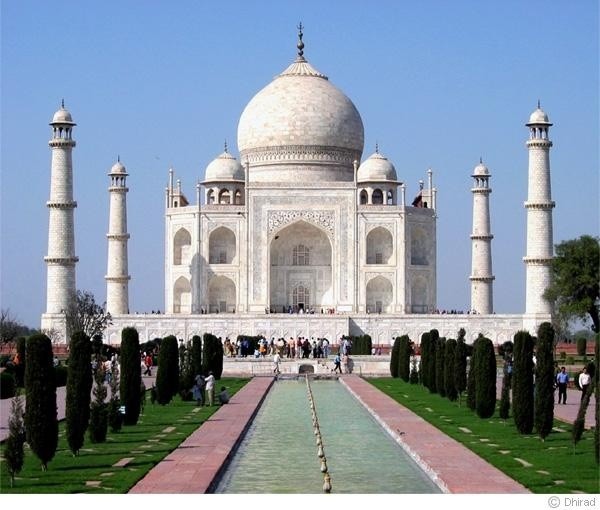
[264,304,335,316]
[409,339,416,358]
[5,344,159,386]
[212,386,230,405]
[202,369,216,408]
[194,369,206,407]
[432,308,485,315]
[175,335,393,374]
[501,346,596,409]
[131,307,223,315]
